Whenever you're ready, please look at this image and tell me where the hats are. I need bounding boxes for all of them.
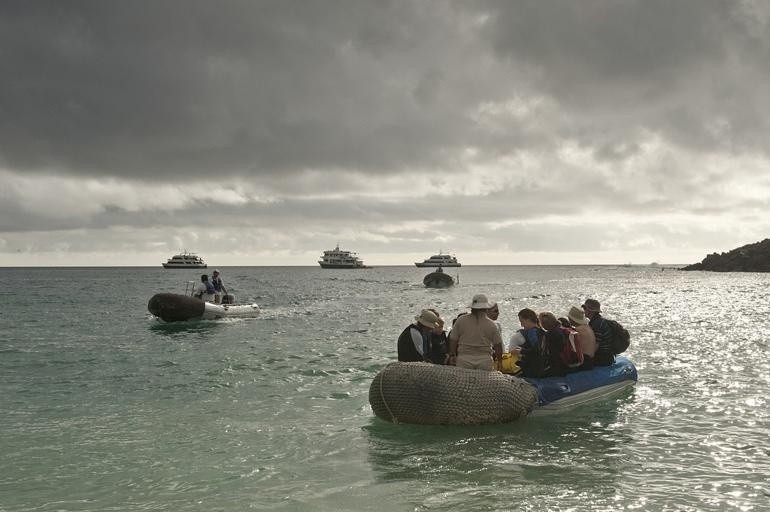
[466,294,496,310]
[414,311,436,330]
[565,306,588,326]
[581,298,602,313]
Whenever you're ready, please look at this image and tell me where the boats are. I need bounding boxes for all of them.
[365,352,639,426]
[318,245,365,268]
[162,249,208,268]
[147,293,261,323]
[423,263,455,287]
[415,249,463,267]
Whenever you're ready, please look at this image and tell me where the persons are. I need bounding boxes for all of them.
[396,294,630,377]
[208,269,228,295]
[193,274,215,304]
[436,264,443,272]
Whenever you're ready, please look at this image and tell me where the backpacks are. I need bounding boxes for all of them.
[599,318,630,355]
